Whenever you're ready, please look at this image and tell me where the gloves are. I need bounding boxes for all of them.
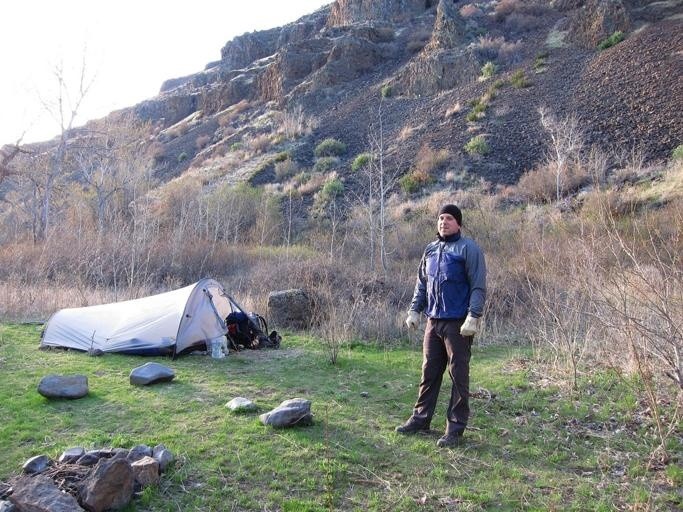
[405,310,421,330]
[460,315,479,338]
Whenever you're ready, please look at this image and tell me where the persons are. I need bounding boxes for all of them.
[395,203,486,446]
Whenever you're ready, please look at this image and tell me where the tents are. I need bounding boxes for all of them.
[41,277,272,361]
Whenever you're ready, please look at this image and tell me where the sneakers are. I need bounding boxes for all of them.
[436,433,464,447]
[396,420,431,433]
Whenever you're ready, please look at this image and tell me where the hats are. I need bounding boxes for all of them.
[437,204,462,227]
